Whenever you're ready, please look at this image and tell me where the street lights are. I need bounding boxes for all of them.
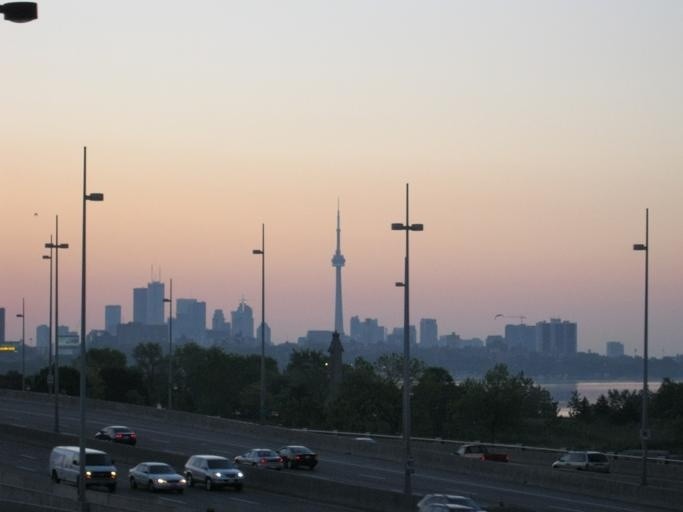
[632,207,653,488]
[251,223,265,425]
[162,277,173,410]
[14,145,111,442]
[389,181,424,495]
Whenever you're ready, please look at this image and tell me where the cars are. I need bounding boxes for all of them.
[127,444,320,494]
[95,425,137,445]
[416,492,490,512]
[455,442,617,473]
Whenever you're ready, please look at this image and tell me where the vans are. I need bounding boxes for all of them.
[48,445,120,493]
